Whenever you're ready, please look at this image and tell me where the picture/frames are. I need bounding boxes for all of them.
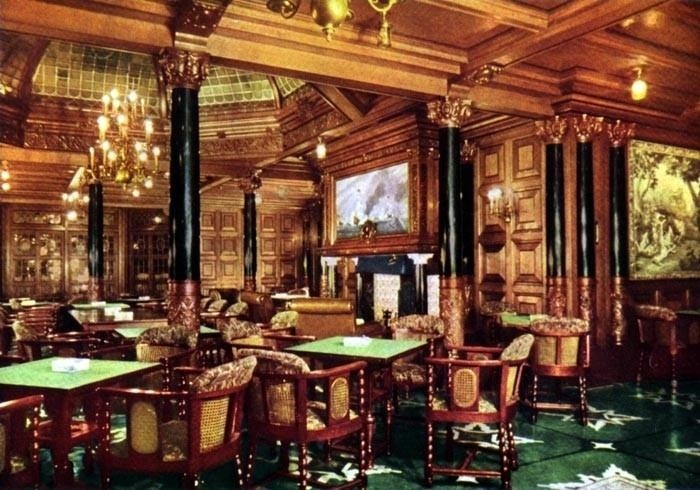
[625,137,700,285]
[332,160,410,246]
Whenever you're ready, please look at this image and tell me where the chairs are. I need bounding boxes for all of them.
[0,293,595,486]
[632,300,699,406]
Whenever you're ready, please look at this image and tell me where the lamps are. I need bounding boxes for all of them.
[265,0,397,49]
[486,189,510,222]
[58,89,161,221]
[629,62,651,103]
[316,138,327,159]
